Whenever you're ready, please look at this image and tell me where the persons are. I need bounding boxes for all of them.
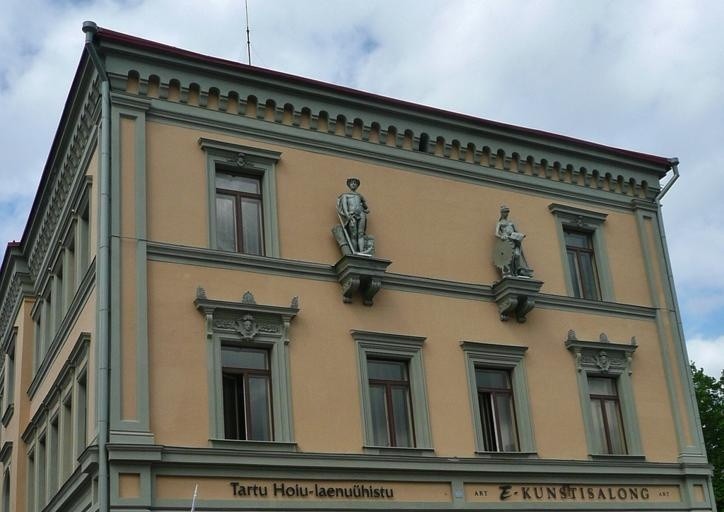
[495,205,534,277]
[335,177,369,255]
[598,351,613,373]
[242,314,253,331]
